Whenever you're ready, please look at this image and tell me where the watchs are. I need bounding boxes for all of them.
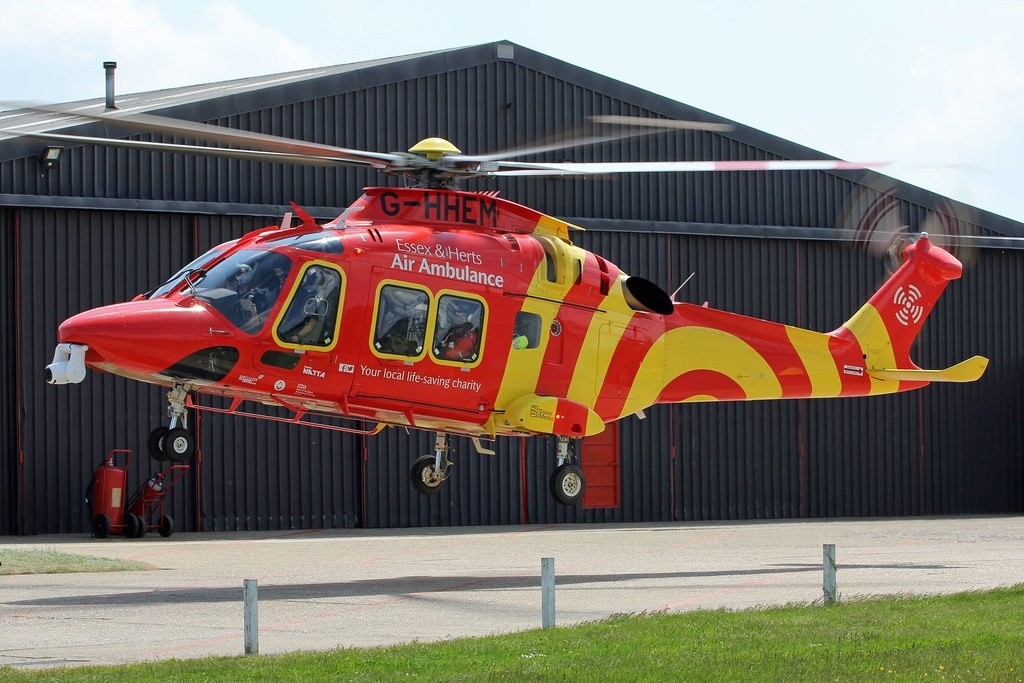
[296,333,303,342]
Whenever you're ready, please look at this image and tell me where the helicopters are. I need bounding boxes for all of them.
[0,101,989,506]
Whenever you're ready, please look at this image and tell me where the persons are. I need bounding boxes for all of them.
[281,260,319,342]
[444,313,478,359]
[235,268,267,314]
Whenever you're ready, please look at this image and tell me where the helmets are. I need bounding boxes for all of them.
[235,264,253,286]
[446,302,472,326]
